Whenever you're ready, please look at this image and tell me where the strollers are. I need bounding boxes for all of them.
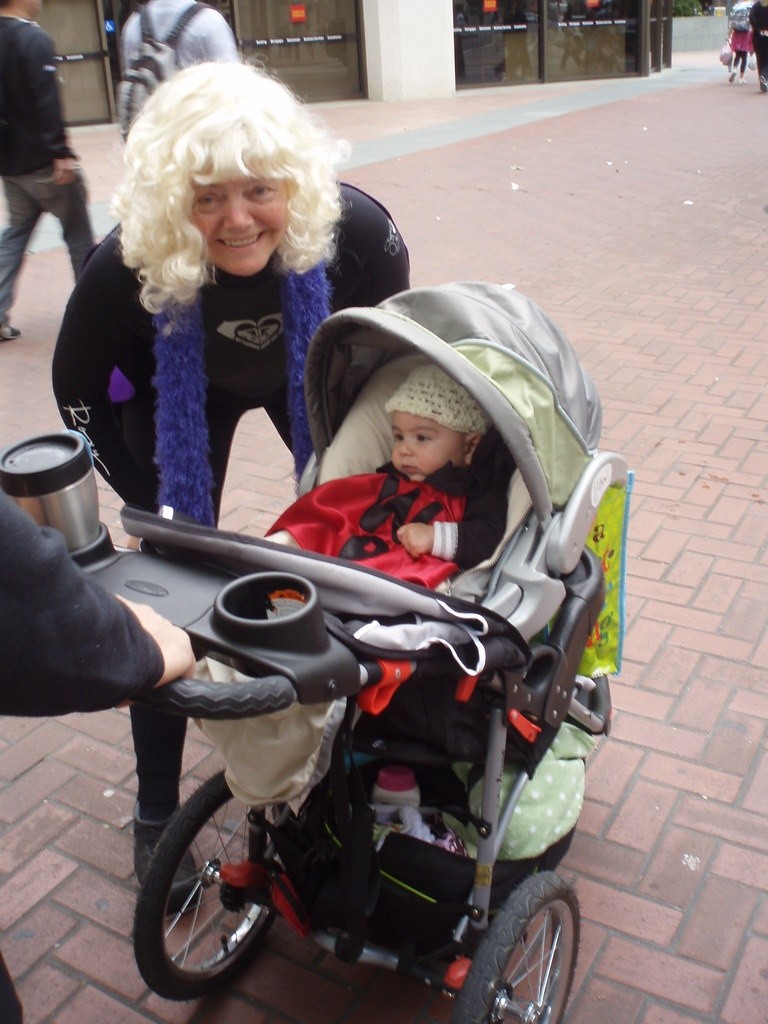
[71,280,634,1024]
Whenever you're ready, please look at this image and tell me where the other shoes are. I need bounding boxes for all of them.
[132,800,200,911]
[0,326,20,338]
[739,79,746,84]
[728,70,736,83]
[760,76,768,92]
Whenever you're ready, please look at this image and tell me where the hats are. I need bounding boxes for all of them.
[386,363,495,437]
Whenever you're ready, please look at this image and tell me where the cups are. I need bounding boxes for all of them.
[0,429,98,552]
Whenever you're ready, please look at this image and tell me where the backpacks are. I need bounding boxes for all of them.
[731,9,750,32]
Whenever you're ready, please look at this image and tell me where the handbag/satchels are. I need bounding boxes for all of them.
[720,40,731,65]
[748,55,757,71]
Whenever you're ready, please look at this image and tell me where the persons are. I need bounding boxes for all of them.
[52,62,412,919]
[719,0,757,84]
[749,0,768,92]
[116,0,242,145]
[0,484,196,1024]
[264,362,508,808]
[0,0,94,339]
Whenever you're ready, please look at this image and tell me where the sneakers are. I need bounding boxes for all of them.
[115,3,221,144]
